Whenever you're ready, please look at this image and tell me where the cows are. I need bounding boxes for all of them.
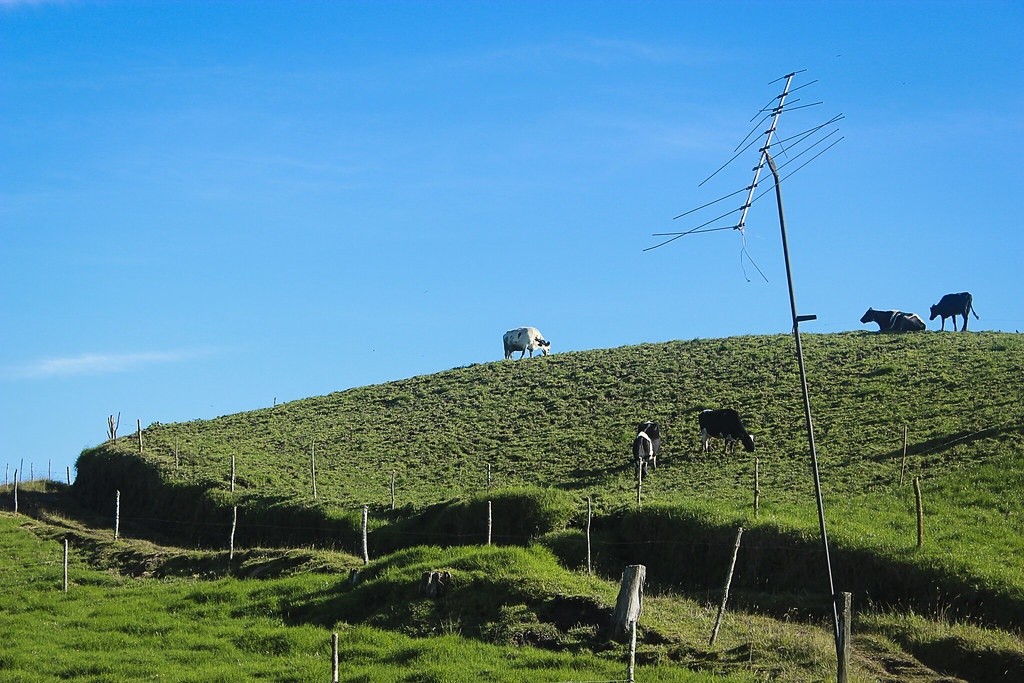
[698,409,757,454]
[632,421,660,481]
[929,292,979,332]
[503,327,551,360]
[860,307,926,333]
